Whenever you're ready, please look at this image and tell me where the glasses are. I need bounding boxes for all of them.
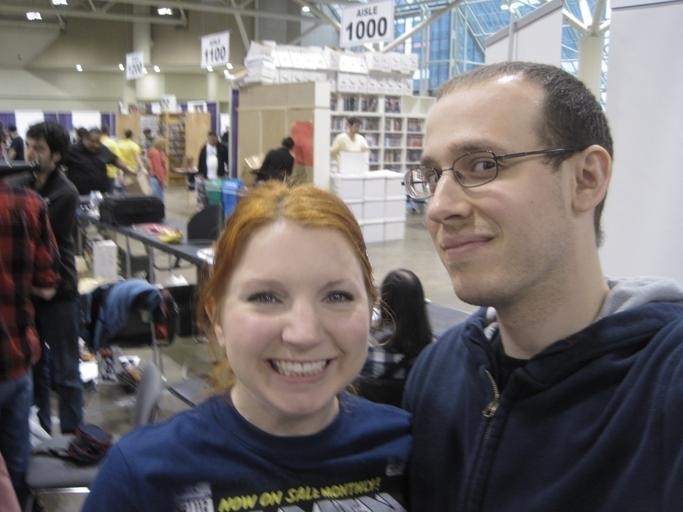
[400,147,563,198]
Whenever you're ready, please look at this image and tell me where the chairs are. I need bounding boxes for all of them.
[25,359,163,512]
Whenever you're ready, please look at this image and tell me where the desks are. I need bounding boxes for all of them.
[76,198,215,278]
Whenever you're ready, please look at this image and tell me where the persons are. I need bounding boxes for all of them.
[256,137,295,185]
[142,128,156,150]
[99,124,117,180]
[146,138,168,192]
[116,129,148,176]
[7,125,23,161]
[355,268,438,404]
[330,117,370,174]
[198,131,228,180]
[4,120,83,436]
[68,128,137,194]
[399,60,683,512]
[80,182,411,512]
[0,128,61,497]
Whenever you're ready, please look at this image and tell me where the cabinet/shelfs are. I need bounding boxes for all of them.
[332,170,407,245]
[330,91,425,173]
